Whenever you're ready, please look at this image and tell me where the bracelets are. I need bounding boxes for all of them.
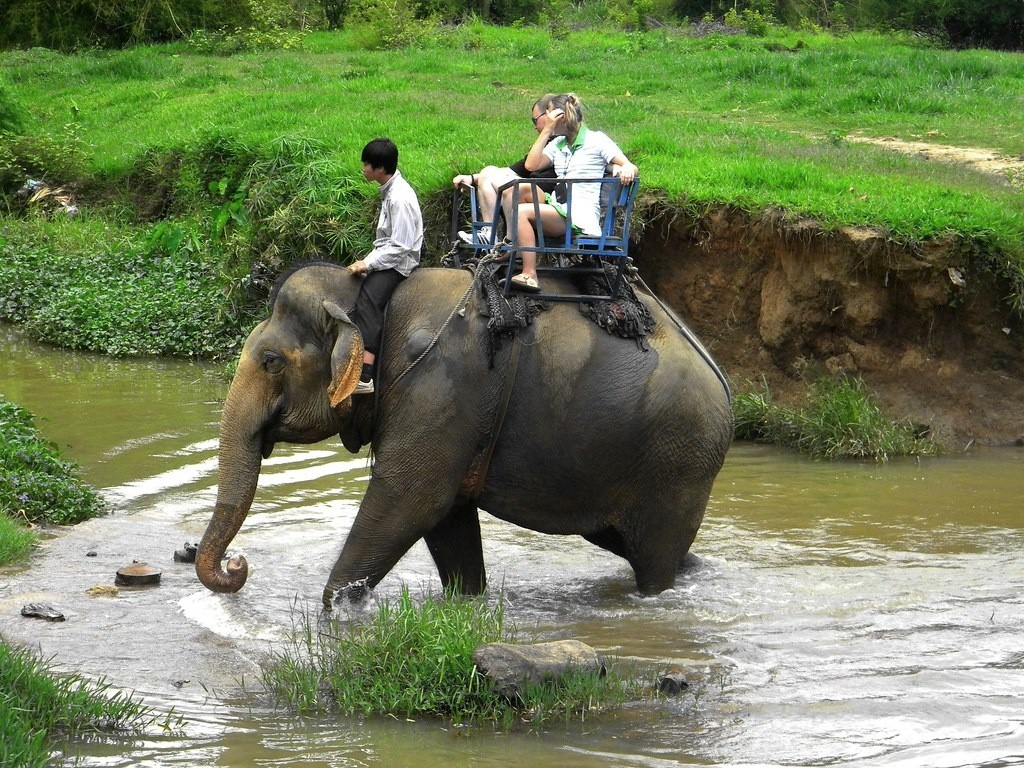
[469,173,474,186]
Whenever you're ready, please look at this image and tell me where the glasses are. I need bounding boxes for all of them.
[532,112,546,124]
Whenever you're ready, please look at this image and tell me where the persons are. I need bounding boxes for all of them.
[345,137,425,396]
[453,93,639,291]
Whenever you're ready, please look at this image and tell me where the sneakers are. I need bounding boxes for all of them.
[457,228,498,250]
[350,379,374,394]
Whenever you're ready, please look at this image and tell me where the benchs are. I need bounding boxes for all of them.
[450,165,641,303]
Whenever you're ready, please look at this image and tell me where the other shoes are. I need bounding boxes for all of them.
[497,273,541,291]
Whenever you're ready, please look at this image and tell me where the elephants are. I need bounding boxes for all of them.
[194,262,737,616]
